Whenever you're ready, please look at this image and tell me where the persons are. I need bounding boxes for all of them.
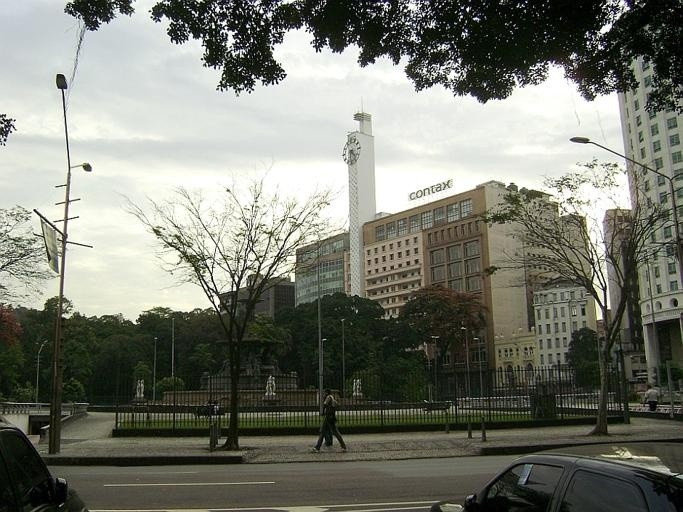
[643,385,659,411]
[312,389,349,453]
[322,395,334,450]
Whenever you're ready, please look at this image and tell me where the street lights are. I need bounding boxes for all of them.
[431,336,440,402]
[460,327,472,439]
[570,136,683,292]
[152,338,158,402]
[322,338,327,391]
[33,74,91,454]
[341,318,346,391]
[473,338,487,442]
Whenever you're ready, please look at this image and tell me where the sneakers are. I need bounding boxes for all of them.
[308,441,348,454]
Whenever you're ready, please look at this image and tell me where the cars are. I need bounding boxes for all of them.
[0,413,89,512]
[429,441,683,512]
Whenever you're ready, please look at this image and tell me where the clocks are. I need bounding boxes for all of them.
[341,137,362,165]
